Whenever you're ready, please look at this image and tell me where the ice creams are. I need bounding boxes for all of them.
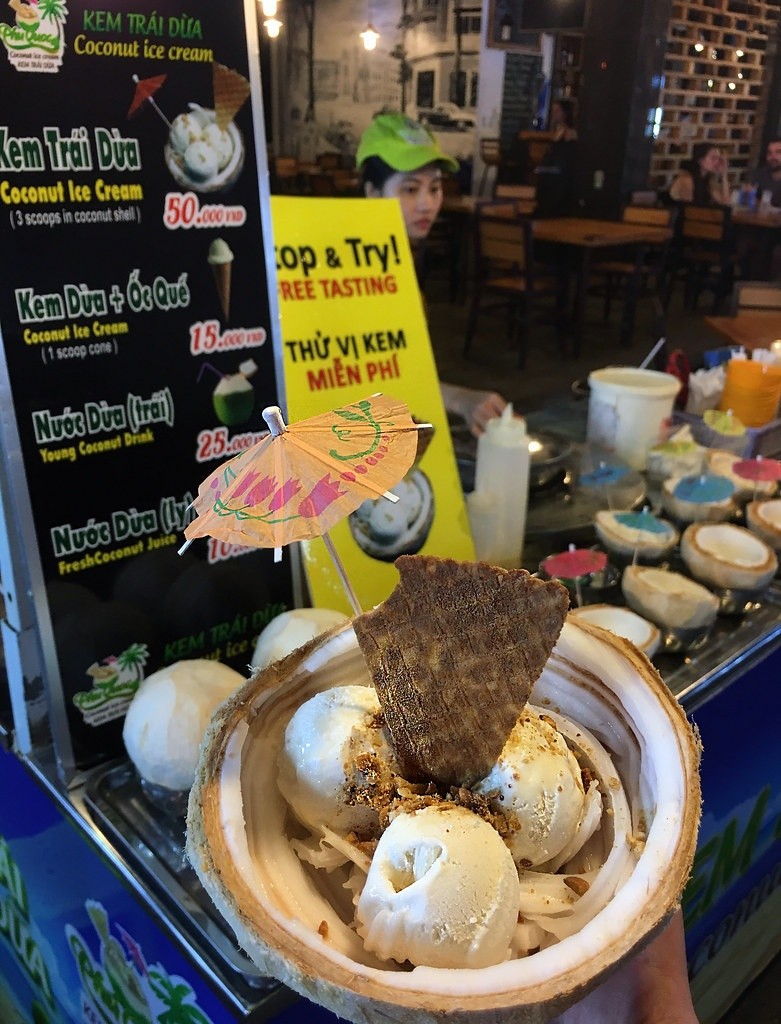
[207,237,234,320]
[168,102,233,181]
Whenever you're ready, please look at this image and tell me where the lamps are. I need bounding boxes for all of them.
[263,16,283,39]
[360,0,381,51]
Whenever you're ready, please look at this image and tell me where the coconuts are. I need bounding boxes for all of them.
[212,374,254,427]
[123,609,706,1024]
[570,448,780,667]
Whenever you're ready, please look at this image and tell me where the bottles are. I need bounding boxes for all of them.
[471,401,530,574]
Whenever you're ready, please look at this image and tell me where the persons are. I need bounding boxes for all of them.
[742,139,781,211]
[352,110,525,441]
[547,99,579,150]
[668,142,730,208]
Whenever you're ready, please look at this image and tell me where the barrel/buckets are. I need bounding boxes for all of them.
[585,369,681,472]
[721,356,781,431]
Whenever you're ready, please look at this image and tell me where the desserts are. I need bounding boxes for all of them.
[275,553,601,965]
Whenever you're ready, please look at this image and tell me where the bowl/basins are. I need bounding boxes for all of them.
[746,497,780,553]
[661,477,736,525]
[619,567,715,632]
[569,605,665,662]
[163,109,245,194]
[680,522,778,590]
[593,508,680,562]
[700,450,779,503]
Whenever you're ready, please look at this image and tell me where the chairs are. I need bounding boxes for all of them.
[272,135,781,384]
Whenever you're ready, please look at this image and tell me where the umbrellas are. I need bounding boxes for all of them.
[178,390,434,616]
[542,406,780,608]
[124,71,171,127]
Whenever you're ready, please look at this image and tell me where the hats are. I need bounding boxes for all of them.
[355,113,460,175]
